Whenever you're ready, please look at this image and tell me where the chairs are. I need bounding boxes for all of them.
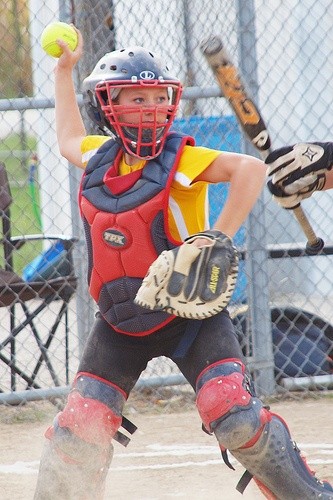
[0,161,79,401]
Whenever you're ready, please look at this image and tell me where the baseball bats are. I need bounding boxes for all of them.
[199,42,326,255]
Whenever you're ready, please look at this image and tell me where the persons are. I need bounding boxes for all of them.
[34,22,333,500]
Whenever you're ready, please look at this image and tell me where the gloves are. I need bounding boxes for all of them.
[266,142,333,210]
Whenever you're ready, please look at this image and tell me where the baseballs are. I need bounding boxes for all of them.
[40,22,78,59]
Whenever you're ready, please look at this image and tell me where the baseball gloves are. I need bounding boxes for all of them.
[135,228,242,322]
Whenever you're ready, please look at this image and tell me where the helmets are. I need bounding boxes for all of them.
[82,46,184,162]
